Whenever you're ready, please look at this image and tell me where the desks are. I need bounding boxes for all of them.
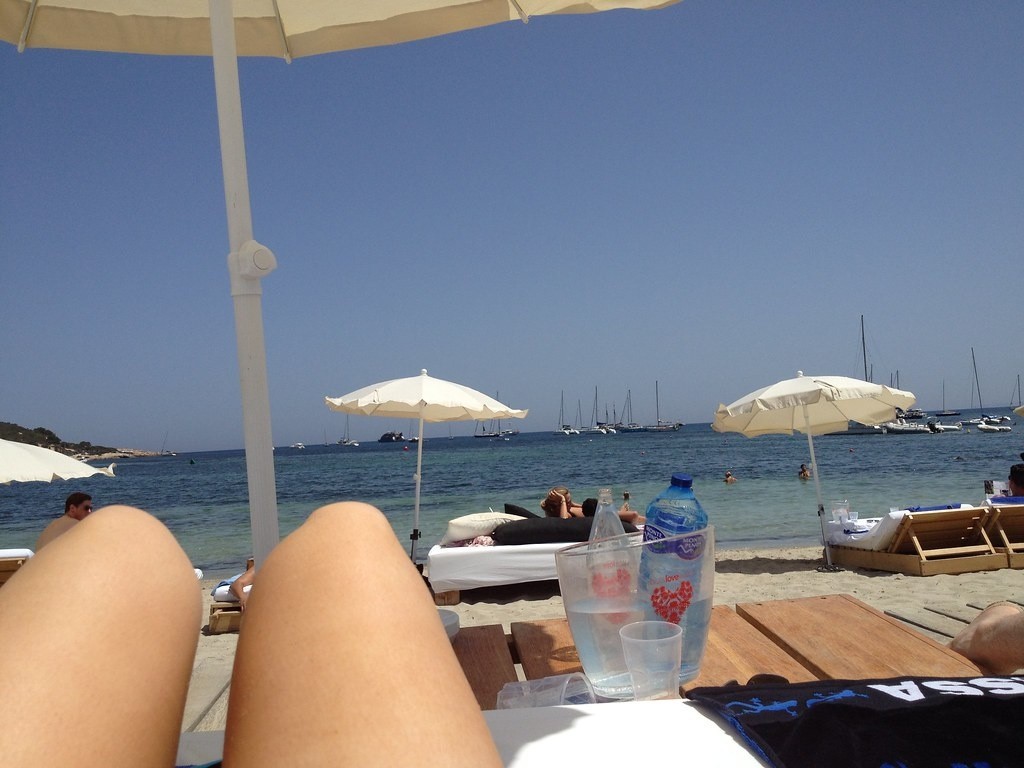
[450,593,997,717]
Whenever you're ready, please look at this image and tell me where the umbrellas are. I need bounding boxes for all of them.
[0,439,117,517]
[1013,405,1024,417]
[0,0,676,573]
[711,371,916,568]
[326,370,528,565]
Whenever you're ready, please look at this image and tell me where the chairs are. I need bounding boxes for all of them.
[819,495,1024,575]
[426,525,646,606]
[0,548,203,586]
[207,584,253,634]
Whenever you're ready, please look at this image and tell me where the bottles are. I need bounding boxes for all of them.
[584,489,646,675]
[636,471,709,609]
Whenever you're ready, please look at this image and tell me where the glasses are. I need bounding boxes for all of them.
[1008,475,1011,481]
[82,505,93,512]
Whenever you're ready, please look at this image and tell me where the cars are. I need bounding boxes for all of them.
[76,455,84,460]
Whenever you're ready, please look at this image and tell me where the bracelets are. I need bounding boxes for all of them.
[560,500,566,504]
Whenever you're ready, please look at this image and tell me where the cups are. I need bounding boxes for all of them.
[618,620,684,700]
[849,512,858,522]
[831,501,850,525]
[497,672,597,710]
[554,524,716,699]
[889,505,899,513]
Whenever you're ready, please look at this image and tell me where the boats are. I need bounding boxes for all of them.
[408,436,420,442]
[291,443,305,449]
[886,418,962,433]
[977,424,1012,433]
[378,432,405,442]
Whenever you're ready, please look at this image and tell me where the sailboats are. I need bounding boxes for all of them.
[1009,374,1024,418]
[161,432,176,456]
[619,390,645,432]
[823,314,887,434]
[890,369,923,419]
[554,390,580,435]
[474,391,519,437]
[336,413,360,446]
[588,386,617,434]
[935,380,961,416]
[645,381,680,431]
[961,346,1003,425]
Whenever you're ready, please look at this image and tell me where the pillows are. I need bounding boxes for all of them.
[439,513,528,546]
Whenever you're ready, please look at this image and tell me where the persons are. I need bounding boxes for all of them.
[540,486,645,525]
[993,464,1024,498]
[798,464,810,480]
[0,493,505,768]
[723,470,736,485]
[945,597,1024,676]
